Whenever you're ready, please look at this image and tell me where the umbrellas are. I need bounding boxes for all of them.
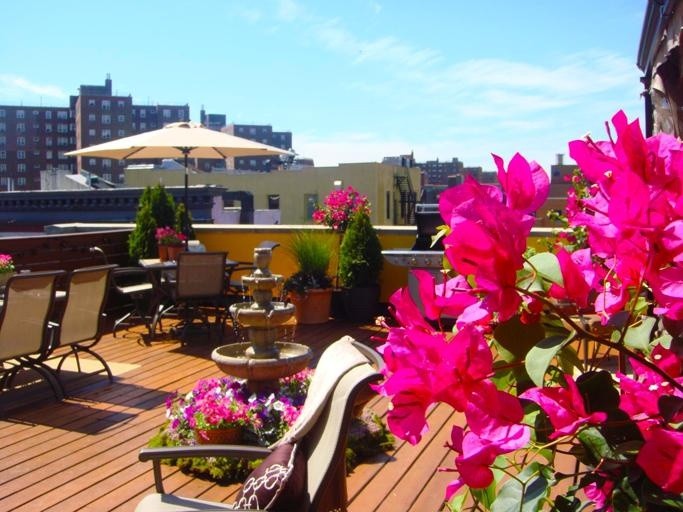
[64,122,301,251]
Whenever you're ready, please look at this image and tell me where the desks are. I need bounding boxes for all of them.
[2,291,68,298]
[139,255,239,339]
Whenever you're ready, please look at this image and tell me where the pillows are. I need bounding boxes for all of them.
[232,440,301,512]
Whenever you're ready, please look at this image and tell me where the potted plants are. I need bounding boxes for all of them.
[336,209,386,326]
[284,226,338,326]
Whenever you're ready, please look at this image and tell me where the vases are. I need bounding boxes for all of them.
[339,220,352,231]
[196,428,240,444]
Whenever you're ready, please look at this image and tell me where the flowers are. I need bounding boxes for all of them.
[156,227,188,244]
[312,185,371,229]
[0,253,17,272]
[245,368,314,444]
[366,109,683,512]
[163,375,262,445]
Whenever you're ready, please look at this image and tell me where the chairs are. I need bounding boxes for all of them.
[135,333,387,512]
[2,270,64,402]
[91,247,158,337]
[173,251,229,345]
[23,263,116,400]
[228,240,281,327]
